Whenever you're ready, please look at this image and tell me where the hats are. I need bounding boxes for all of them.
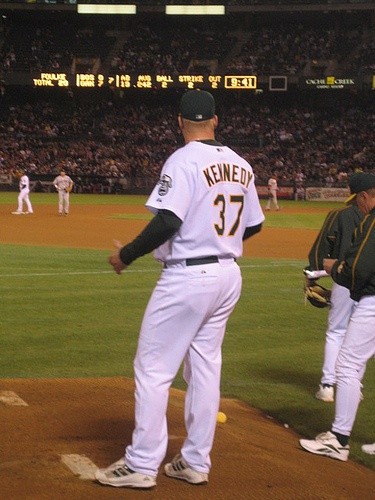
[59,168,66,171]
[344,172,375,205]
[178,88,216,121]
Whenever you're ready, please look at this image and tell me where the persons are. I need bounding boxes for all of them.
[94,88,265,487]
[10,170,34,215]
[304,202,365,402]
[53,169,73,217]
[297,172,374,462]
[266,173,280,210]
[2,25,373,182]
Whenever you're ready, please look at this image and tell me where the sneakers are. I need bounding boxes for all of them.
[315,383,336,403]
[95,454,157,490]
[58,211,61,215]
[26,210,31,214]
[297,432,351,463]
[163,454,210,486]
[15,210,20,211]
[360,443,374,452]
[65,213,66,215]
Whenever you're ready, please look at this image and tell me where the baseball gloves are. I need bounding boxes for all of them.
[306,280,332,309]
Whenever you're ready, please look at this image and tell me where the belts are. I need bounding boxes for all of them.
[162,257,238,266]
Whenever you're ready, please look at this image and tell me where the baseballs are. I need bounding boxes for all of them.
[216,411,227,423]
